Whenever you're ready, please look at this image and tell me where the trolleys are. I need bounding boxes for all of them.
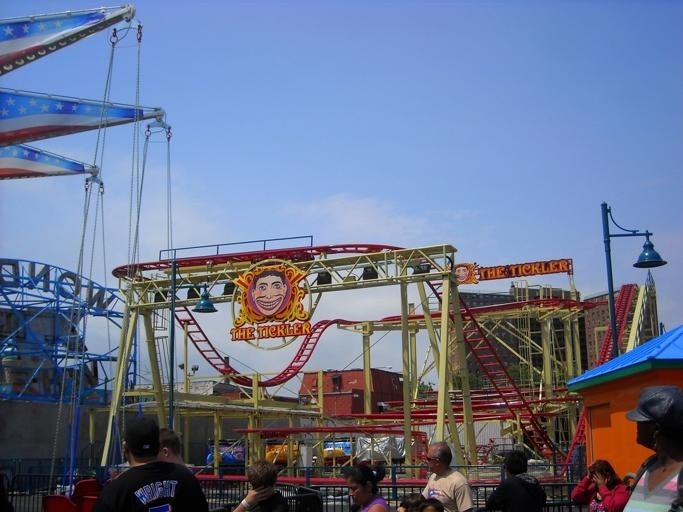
[489,450,506,465]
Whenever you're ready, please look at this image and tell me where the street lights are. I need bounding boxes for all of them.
[168,261,217,431]
[600,200,668,360]
[176,361,198,464]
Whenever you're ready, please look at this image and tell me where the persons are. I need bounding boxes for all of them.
[94,413,472,511]
[452,264,470,282]
[485,386,680,511]
[250,271,288,317]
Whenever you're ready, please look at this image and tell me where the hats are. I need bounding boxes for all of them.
[125,416,159,450]
[625,386,683,423]
